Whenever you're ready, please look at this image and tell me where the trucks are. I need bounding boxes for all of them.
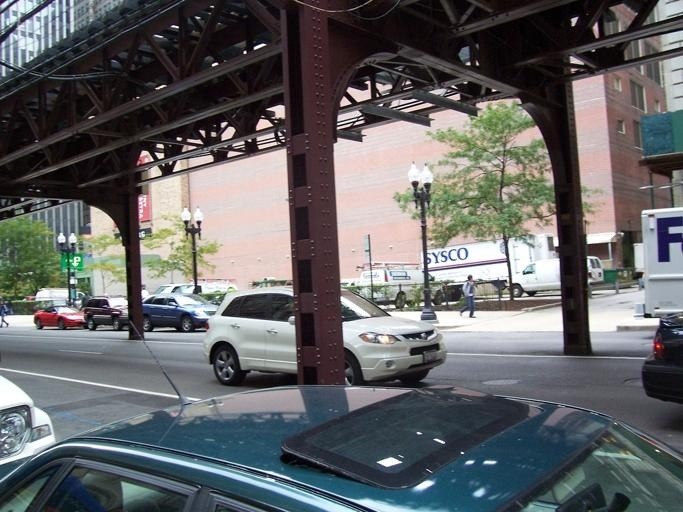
[420,234,557,301]
[641,207,683,318]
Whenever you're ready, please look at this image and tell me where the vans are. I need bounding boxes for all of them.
[506,255,604,297]
[83,297,129,331]
[153,282,236,299]
[360,270,447,308]
[34,288,79,309]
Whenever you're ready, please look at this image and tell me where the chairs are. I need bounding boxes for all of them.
[50,471,123,512]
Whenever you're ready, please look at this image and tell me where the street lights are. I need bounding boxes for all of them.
[408,161,434,290]
[58,232,78,306]
[181,206,203,294]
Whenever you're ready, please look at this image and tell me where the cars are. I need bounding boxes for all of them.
[641,309,682,403]
[1,374,57,481]
[35,304,88,329]
[142,294,219,332]
[1,385,683,508]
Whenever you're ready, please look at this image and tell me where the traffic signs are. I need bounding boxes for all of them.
[60,253,85,274]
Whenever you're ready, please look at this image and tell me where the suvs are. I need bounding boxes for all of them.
[202,285,446,387]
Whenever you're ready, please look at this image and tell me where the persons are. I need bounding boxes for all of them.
[0,300,8,328]
[141,285,150,299]
[459,275,476,318]
[82,291,90,305]
